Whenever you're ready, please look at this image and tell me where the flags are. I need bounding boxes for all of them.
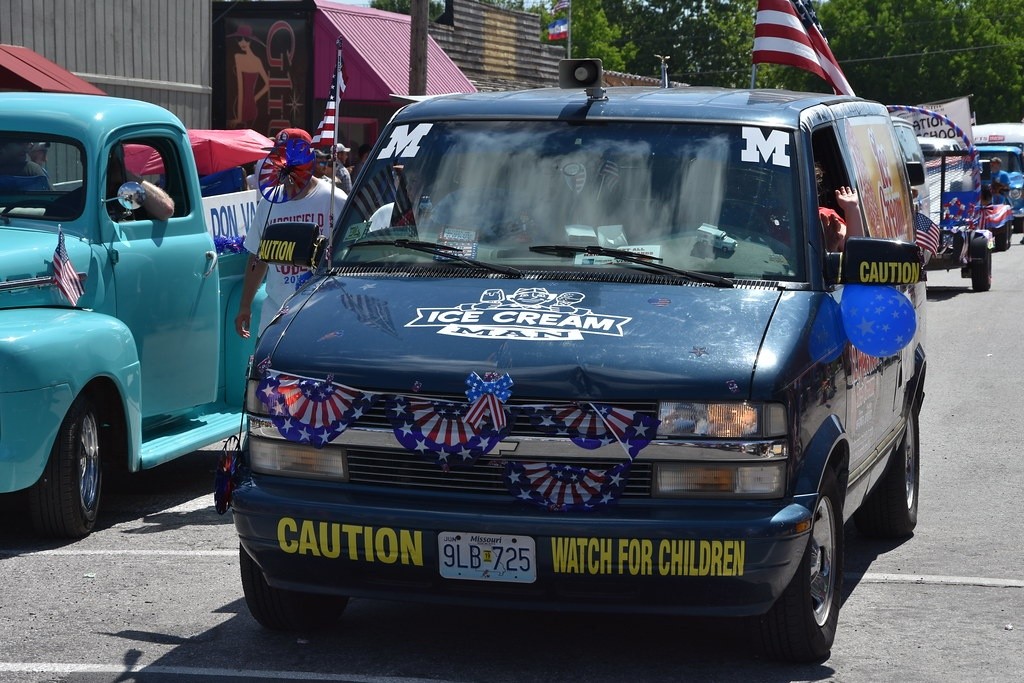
[554,0,570,11]
[915,211,940,258]
[752,0,858,96]
[311,40,348,148]
[51,232,85,306]
[548,16,569,39]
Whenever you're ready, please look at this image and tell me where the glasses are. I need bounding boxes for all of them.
[315,160,328,167]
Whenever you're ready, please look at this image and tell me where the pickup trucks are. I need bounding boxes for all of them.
[0,88,269,538]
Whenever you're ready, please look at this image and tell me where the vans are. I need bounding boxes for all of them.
[220,83,930,666]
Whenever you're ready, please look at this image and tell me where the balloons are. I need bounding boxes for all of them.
[839,281,918,359]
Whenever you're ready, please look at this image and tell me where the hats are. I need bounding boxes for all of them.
[314,149,329,157]
[260,129,312,156]
[330,143,351,152]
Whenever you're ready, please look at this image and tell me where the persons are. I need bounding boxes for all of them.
[987,157,1012,204]
[313,142,372,194]
[367,161,567,249]
[1020,152,1024,244]
[774,161,866,253]
[47,151,175,221]
[0,138,54,191]
[236,126,349,351]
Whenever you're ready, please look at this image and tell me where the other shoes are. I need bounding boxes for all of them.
[1020,237,1024,244]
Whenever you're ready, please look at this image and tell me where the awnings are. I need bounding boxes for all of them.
[0,44,108,95]
[314,5,480,103]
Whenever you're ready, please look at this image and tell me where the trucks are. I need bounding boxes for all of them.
[890,115,1024,291]
